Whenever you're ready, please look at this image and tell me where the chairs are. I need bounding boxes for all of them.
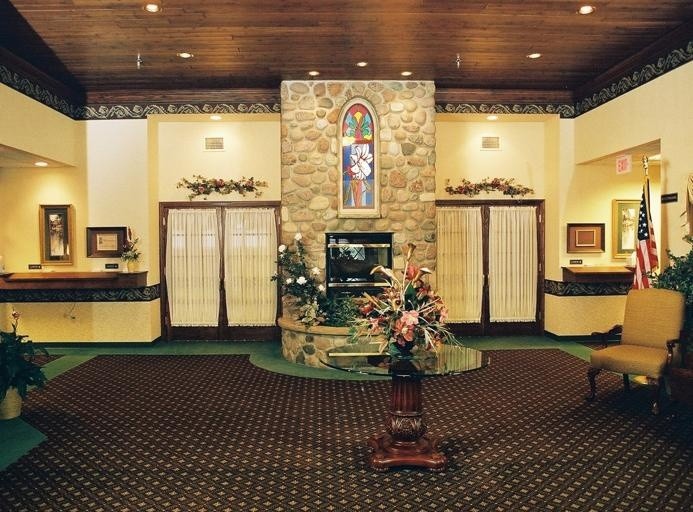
[585,285,688,415]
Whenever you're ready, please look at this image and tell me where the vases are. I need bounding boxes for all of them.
[387,336,419,361]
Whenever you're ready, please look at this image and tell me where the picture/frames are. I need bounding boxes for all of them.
[610,197,642,260]
[334,95,384,222]
[565,221,606,255]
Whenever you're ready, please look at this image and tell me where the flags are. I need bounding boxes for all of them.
[631,185,657,290]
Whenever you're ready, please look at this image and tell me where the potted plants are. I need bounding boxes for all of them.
[0,322,53,424]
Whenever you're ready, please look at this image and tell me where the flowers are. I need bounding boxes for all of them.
[441,174,536,200]
[268,231,352,327]
[175,173,266,204]
[84,224,130,259]
[37,203,76,267]
[349,240,464,355]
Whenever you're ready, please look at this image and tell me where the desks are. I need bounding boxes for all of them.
[559,263,634,282]
[313,340,495,475]
